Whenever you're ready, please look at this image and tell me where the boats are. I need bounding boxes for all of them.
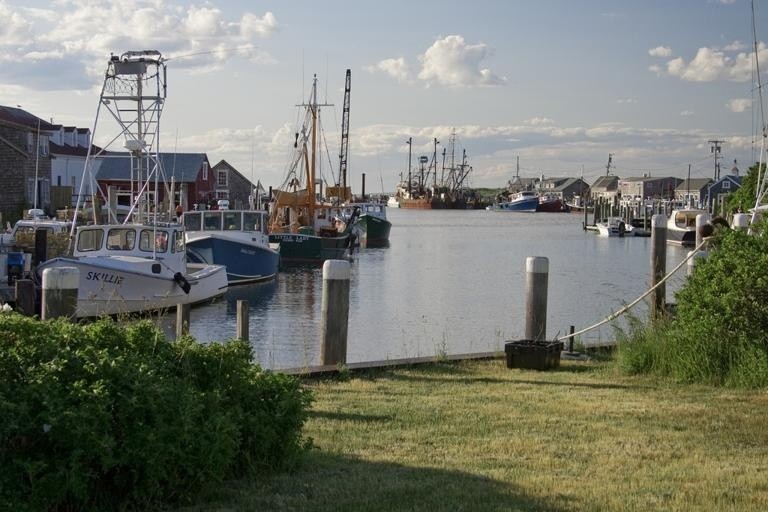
[596,217,635,237]
[175,200,282,284]
[495,191,562,212]
[667,209,708,245]
[25,48,228,321]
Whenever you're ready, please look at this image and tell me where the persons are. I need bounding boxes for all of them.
[712,217,729,229]
[700,224,714,237]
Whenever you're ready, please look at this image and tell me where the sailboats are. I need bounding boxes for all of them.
[241,68,395,262]
[394,128,480,209]
[8,117,76,253]
